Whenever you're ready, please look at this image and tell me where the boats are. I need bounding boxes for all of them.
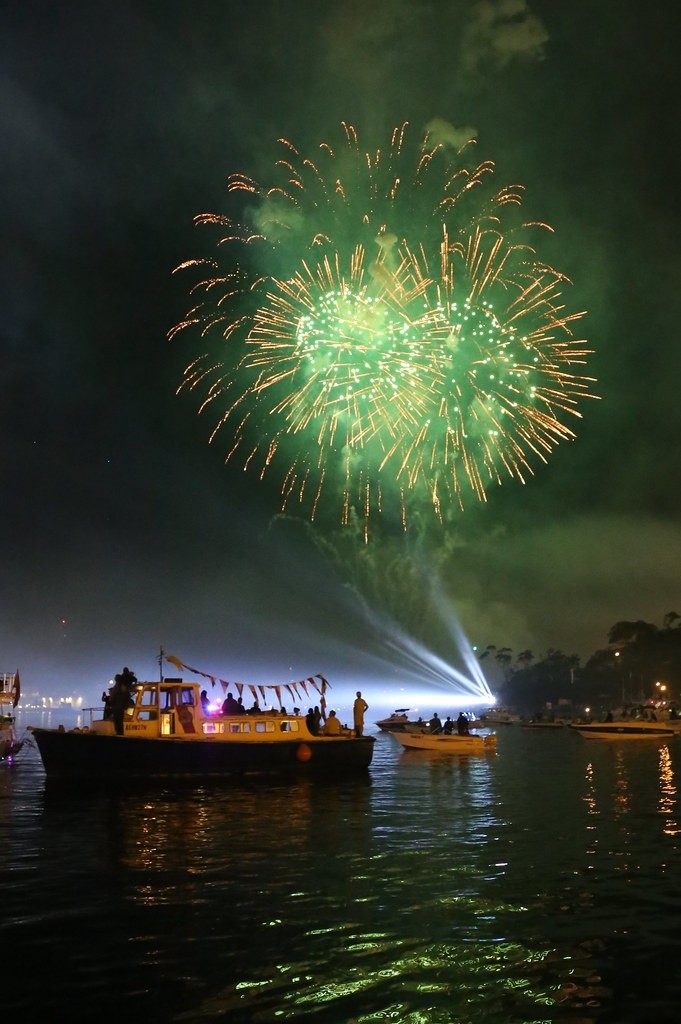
[478,707,521,726]
[450,720,486,729]
[402,723,433,734]
[569,720,676,739]
[386,728,497,753]
[373,708,432,732]
[25,646,377,786]
[0,667,27,763]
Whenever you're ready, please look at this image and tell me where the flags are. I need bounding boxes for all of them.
[12,671,20,708]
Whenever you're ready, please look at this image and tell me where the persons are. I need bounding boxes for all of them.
[457,712,469,735]
[102,666,136,736]
[353,691,369,737]
[222,693,245,714]
[251,701,261,712]
[430,713,442,735]
[418,717,422,722]
[604,713,613,722]
[294,708,300,716]
[280,707,287,731]
[58,724,89,734]
[647,711,657,722]
[305,706,321,736]
[444,716,452,735]
[322,710,342,736]
[201,690,210,715]
